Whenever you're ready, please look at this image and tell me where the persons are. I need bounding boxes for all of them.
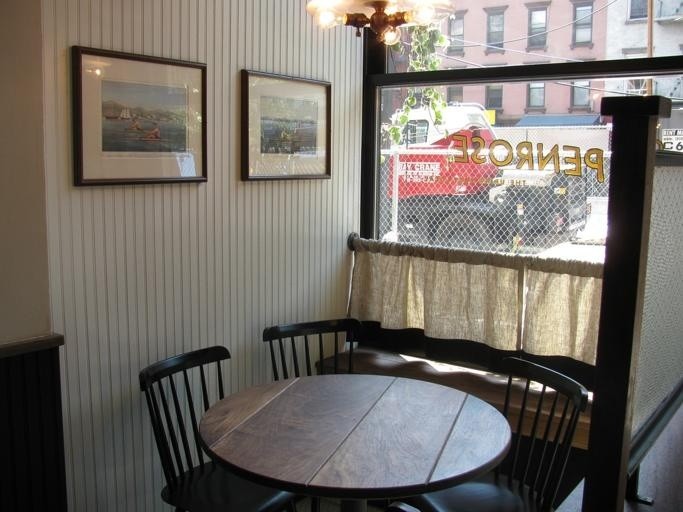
[141,122,161,139]
[280,128,289,138]
[124,119,143,132]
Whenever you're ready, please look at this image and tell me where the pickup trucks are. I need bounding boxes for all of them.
[380,126,588,252]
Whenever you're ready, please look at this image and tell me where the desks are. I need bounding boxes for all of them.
[198,372,513,511]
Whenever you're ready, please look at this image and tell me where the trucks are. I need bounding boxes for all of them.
[379,103,612,196]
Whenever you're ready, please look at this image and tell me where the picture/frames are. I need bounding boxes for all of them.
[70,42,208,187]
[240,64,332,183]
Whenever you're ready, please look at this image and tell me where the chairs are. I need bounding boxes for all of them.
[138,345,308,511]
[262,317,362,381]
[422,356,590,511]
